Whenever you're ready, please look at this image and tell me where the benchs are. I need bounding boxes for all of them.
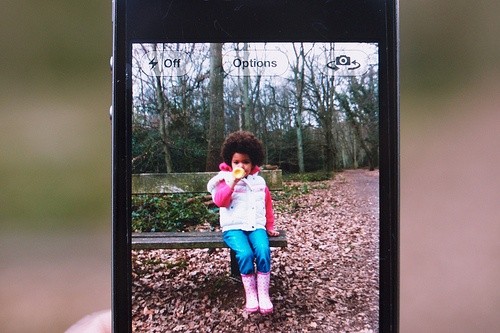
[131,170,288,277]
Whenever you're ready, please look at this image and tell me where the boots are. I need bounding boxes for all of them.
[241,269,273,315]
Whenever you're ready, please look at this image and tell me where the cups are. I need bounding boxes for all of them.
[233,167,245,178]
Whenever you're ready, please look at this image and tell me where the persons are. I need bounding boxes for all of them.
[206,130,280,313]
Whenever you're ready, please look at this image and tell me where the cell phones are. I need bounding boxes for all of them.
[112,0,401,333]
